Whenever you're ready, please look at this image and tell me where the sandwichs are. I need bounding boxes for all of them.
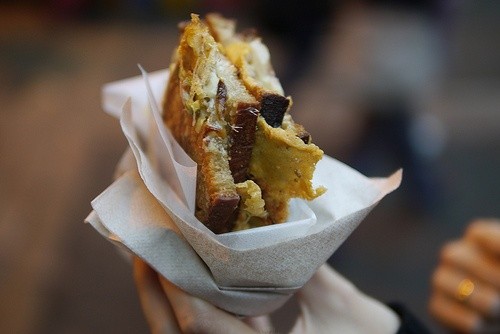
[158,12,327,234]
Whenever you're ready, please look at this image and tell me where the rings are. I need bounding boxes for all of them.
[453,274,475,303]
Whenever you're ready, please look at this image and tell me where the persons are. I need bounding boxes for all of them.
[132,216,500,334]
